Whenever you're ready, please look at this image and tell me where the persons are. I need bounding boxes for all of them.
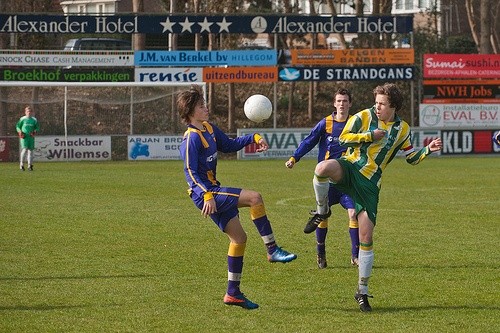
[16,106,39,171]
[286,88,363,269]
[304,83,441,312]
[177,89,298,311]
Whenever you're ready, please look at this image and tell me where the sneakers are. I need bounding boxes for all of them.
[354,293,373,313]
[224,292,259,310]
[351,257,359,266]
[317,254,327,269]
[20,165,25,171]
[267,246,297,263]
[304,208,331,234]
[28,165,33,171]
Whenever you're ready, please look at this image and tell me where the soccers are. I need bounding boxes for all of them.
[243,94,273,123]
